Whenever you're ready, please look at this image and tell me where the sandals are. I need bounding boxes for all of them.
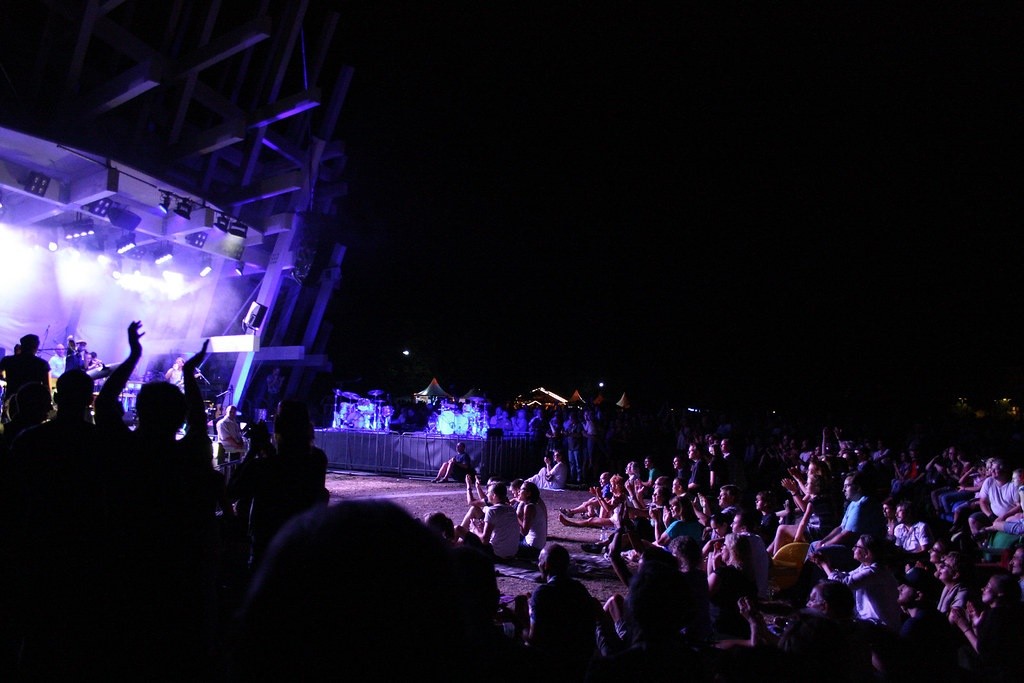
[559,507,574,518]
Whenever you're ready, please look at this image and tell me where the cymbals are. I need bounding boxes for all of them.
[470,397,482,402]
[376,399,386,403]
[332,387,343,396]
[342,392,361,400]
[447,403,458,408]
[369,389,384,396]
[92,364,120,380]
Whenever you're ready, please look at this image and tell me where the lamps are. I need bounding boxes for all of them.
[190,232,208,247]
[89,198,112,218]
[172,202,191,220]
[115,233,136,253]
[25,170,50,198]
[153,244,173,264]
[63,218,95,240]
[200,258,211,277]
[211,213,249,239]
[159,195,171,214]
[236,260,245,276]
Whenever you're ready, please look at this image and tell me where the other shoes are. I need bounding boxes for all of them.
[580,544,602,554]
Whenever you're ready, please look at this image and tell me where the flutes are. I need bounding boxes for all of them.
[195,367,211,385]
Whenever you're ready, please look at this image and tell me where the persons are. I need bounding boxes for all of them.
[431,442,471,484]
[529,416,1024,683]
[459,475,547,563]
[490,405,627,484]
[523,543,601,682]
[0,320,501,683]
[524,449,568,489]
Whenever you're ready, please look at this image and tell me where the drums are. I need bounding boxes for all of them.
[434,404,490,438]
[116,391,137,413]
[91,392,100,415]
[338,398,394,430]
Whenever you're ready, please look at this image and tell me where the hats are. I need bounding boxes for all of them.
[894,567,938,599]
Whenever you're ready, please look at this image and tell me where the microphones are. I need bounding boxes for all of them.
[45,324,50,332]
[231,385,234,391]
